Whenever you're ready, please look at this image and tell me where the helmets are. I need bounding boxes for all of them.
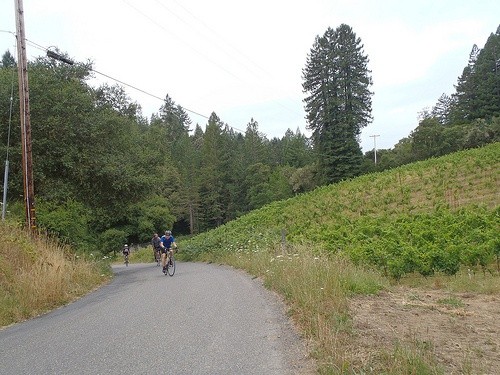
[124,245,128,246]
[154,233,158,236]
[165,230,172,235]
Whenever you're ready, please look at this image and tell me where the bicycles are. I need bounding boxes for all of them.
[162,247,180,276]
[123,252,130,266]
[153,247,161,266]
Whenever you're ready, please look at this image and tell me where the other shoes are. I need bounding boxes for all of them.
[163,269,166,273]
[170,261,173,264]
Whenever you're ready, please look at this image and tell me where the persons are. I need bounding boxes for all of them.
[152,234,162,262]
[123,245,130,264]
[159,230,177,272]
